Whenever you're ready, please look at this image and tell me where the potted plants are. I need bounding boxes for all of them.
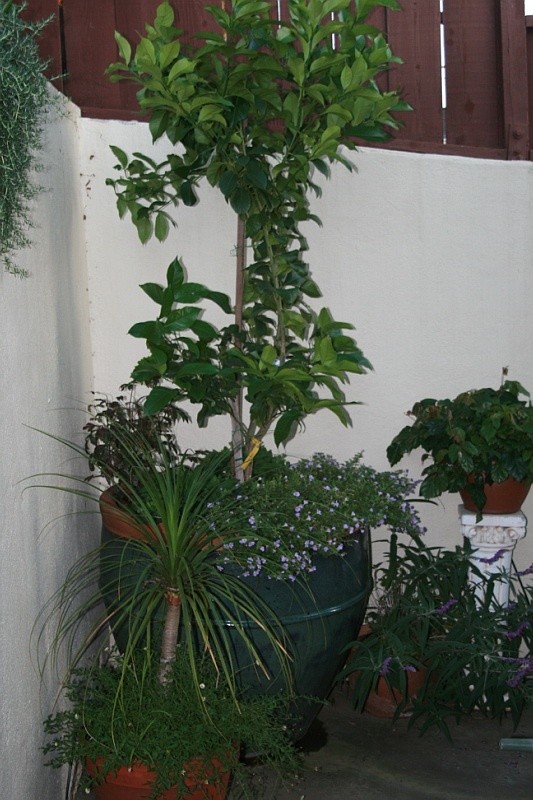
[386,377,533,513]
[18,424,301,799]
[348,608,432,720]
[95,0,414,752]
